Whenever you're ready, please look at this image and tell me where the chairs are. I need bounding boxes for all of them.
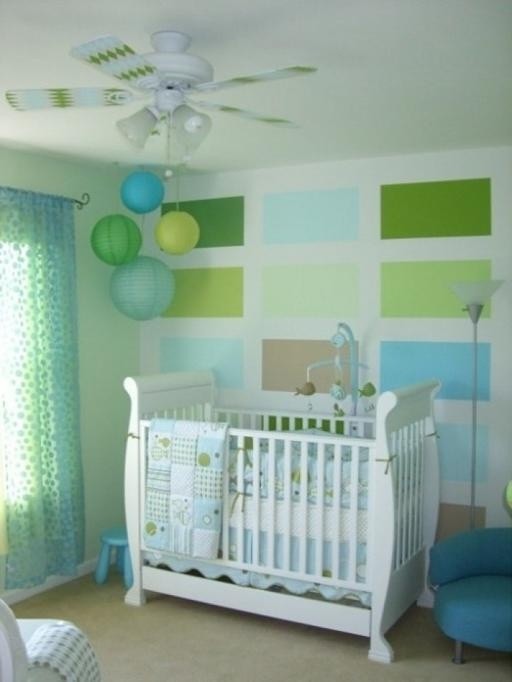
[0,599,100,682]
[427,528,512,664]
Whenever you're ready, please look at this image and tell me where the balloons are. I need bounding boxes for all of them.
[155,211,200,255]
[120,171,164,214]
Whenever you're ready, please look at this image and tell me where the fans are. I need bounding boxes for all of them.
[6,31,317,140]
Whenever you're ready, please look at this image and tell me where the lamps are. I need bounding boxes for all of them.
[116,89,211,178]
[449,281,502,527]
[91,170,200,320]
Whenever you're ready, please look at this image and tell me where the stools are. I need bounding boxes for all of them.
[94,524,133,589]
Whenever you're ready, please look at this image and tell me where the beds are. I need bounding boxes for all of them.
[123,372,441,663]
